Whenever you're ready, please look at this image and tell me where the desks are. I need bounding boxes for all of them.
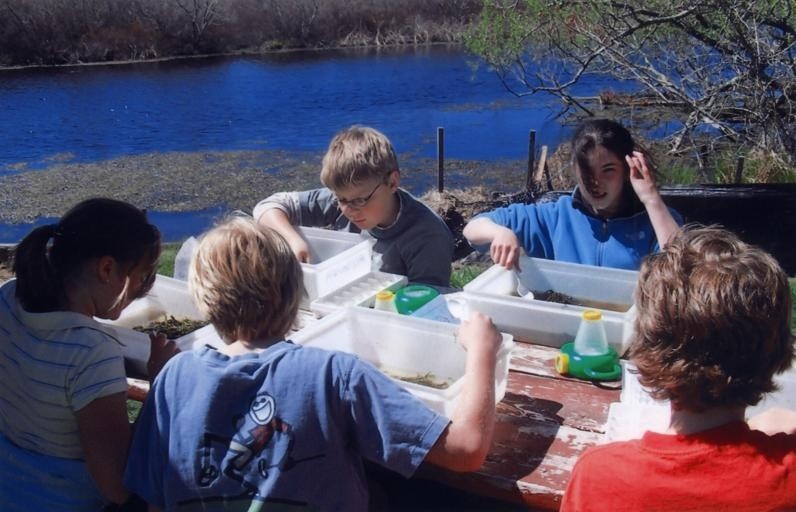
[126,341,796,511]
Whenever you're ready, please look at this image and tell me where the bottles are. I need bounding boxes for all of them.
[374,289,399,315]
[572,308,611,357]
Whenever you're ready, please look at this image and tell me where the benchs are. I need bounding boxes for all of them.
[657,182,795,278]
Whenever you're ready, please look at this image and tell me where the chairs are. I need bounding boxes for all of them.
[0,434,115,511]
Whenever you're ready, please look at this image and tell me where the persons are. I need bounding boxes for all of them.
[251,122,455,287]
[0,196,184,511]
[461,118,691,274]
[122,209,505,511]
[557,219,796,512]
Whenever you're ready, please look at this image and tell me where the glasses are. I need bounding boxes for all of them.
[328,180,383,208]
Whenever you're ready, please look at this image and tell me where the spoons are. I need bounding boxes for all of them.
[511,265,536,300]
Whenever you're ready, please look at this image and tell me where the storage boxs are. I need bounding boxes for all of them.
[193,224,379,307]
[92,272,224,379]
[285,305,515,422]
[462,253,640,358]
